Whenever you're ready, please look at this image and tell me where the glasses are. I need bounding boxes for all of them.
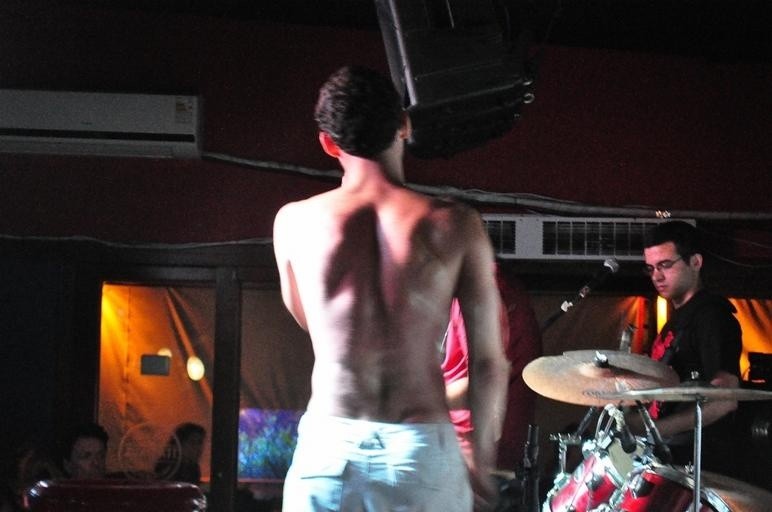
[641,255,684,275]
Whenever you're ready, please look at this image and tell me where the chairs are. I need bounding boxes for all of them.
[21,475,209,512]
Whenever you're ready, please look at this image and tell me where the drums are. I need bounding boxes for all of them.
[542,438,663,512]
[609,462,728,512]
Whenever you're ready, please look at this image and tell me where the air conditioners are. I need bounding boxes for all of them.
[0,86,202,161]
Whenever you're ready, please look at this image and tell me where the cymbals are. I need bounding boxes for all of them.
[523,349,681,409]
[588,381,768,403]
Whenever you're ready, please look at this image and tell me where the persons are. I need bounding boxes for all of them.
[615,221,744,479]
[273,61,511,512]
[155,423,205,484]
[440,267,541,512]
[53,420,109,482]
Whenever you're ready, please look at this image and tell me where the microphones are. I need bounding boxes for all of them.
[570,256,619,305]
